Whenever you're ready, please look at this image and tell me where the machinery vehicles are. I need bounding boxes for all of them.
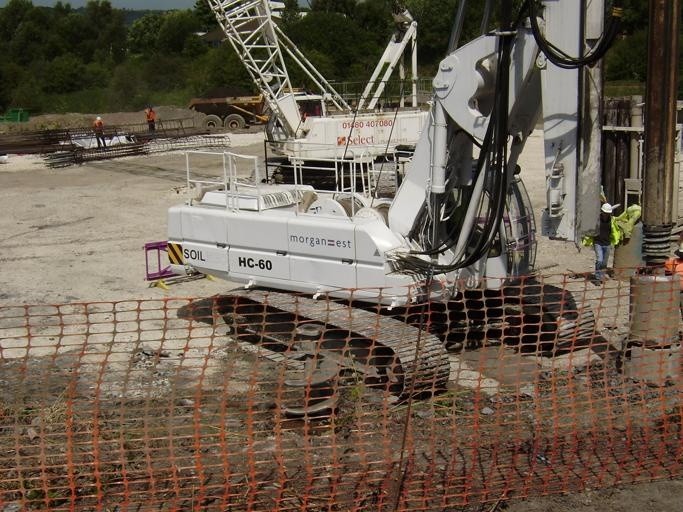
[142,1,681,407]
[210,2,433,197]
[187,85,312,132]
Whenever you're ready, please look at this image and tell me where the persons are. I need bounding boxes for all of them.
[90,115,106,148]
[615,202,641,246]
[143,103,155,133]
[663,242,683,340]
[604,203,623,275]
[579,202,626,286]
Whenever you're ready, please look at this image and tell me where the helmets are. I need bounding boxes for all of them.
[601,203,612,214]
